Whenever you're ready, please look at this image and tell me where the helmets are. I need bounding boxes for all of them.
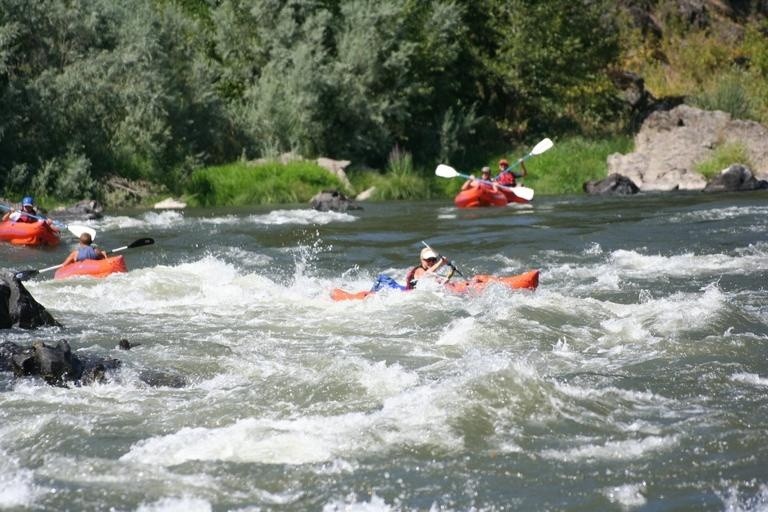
[22,197,33,207]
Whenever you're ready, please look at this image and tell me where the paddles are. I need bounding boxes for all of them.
[1,205,96,241]
[490,137,553,182]
[435,164,534,201]
[13,237,154,282]
[422,240,469,282]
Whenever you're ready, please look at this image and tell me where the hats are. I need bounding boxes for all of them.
[481,167,490,173]
[499,159,508,164]
[420,248,439,260]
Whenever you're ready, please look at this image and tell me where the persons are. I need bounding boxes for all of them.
[494,158,527,187]
[405,247,457,290]
[461,166,498,194]
[2,196,37,222]
[63,232,107,266]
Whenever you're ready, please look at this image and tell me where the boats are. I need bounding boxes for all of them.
[454,183,507,208]
[501,186,529,205]
[1,241,56,262]
[1,212,60,246]
[332,270,540,302]
[53,255,128,278]
